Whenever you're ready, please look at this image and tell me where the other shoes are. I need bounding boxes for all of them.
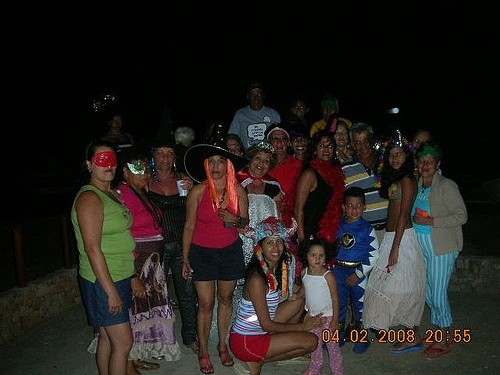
[134,359,160,370]
[186,339,199,354]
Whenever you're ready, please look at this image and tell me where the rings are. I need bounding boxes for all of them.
[113,309,114,312]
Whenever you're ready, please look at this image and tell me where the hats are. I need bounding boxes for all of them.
[264,122,293,140]
[153,133,177,152]
[247,79,266,94]
[244,216,299,242]
[246,142,277,170]
[184,140,248,183]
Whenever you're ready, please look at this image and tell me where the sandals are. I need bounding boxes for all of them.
[198,352,215,374]
[218,348,235,367]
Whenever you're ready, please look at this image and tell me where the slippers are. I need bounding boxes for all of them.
[423,340,453,359]
[387,337,424,354]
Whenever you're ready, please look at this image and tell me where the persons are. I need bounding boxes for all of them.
[363,140,422,352]
[71,141,137,374]
[182,143,250,372]
[410,146,467,356]
[286,236,344,375]
[86,78,432,352]
[114,156,181,370]
[228,217,327,375]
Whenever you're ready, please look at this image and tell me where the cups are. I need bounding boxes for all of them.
[176,179,189,196]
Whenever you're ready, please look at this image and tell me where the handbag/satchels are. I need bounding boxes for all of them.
[86,295,181,362]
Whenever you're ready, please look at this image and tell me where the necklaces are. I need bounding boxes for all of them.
[211,187,227,205]
